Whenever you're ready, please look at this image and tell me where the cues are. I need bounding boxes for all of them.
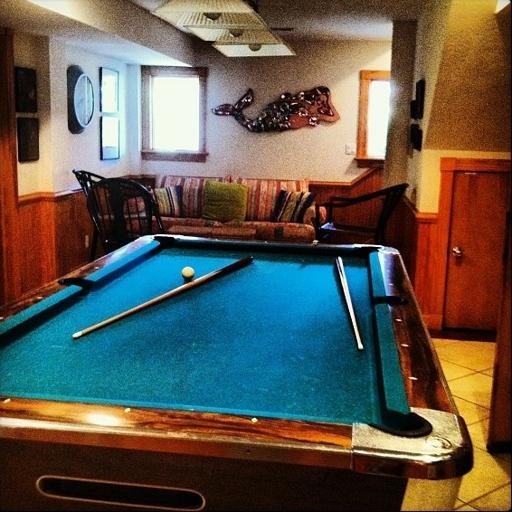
[73,255,253,339]
[336,256,364,350]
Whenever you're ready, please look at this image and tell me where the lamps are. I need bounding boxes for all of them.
[128,0,298,58]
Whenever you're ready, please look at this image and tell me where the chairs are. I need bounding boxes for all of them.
[86,179,154,251]
[316,182,410,244]
[72,168,168,259]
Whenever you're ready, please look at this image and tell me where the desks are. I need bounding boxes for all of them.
[167,224,258,242]
[0,234,475,512]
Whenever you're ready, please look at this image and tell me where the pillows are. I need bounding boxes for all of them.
[272,189,316,223]
[201,179,248,219]
[125,184,182,219]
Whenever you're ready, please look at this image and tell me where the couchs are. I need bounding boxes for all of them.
[126,171,327,242]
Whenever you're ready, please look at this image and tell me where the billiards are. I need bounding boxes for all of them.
[182,266,195,278]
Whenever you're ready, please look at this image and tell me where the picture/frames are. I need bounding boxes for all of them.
[99,66,119,113]
[100,116,120,160]
